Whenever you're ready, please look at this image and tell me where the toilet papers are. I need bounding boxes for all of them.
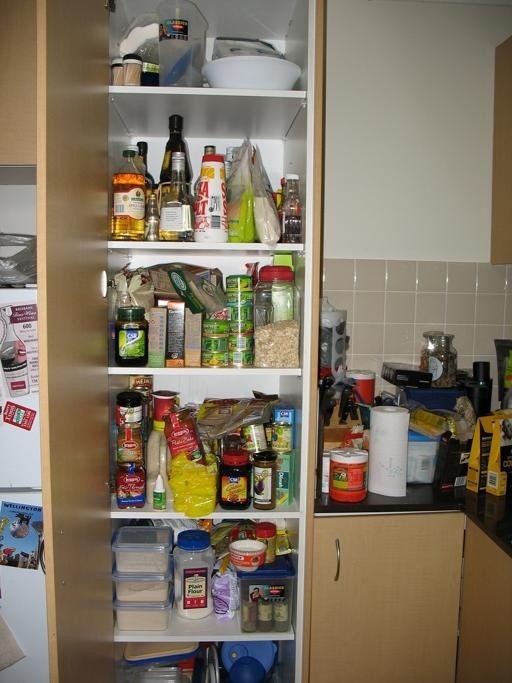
[368,406,410,496]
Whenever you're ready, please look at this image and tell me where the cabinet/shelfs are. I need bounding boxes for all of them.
[309,512,466,682]
[35,1,323,682]
[455,518,511,683]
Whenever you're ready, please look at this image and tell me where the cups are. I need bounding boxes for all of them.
[228,540,267,571]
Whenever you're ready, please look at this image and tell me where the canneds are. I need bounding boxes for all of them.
[162,409,206,467]
[253,452,278,510]
[219,452,253,509]
[201,275,253,368]
[243,422,268,454]
[109,174,145,242]
[129,375,153,402]
[116,307,149,368]
[116,422,145,510]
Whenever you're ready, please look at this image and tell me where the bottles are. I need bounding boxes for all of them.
[173,530,214,619]
[153,474,166,511]
[0,305,31,398]
[253,266,300,369]
[243,596,289,631]
[420,331,458,388]
[122,53,143,85]
[109,58,124,85]
[109,115,303,242]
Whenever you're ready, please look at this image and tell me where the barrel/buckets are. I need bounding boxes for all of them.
[145,418,174,504]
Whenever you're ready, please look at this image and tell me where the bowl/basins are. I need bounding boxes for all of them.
[201,55,301,90]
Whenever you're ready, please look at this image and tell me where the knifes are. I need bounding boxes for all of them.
[320,376,353,425]
